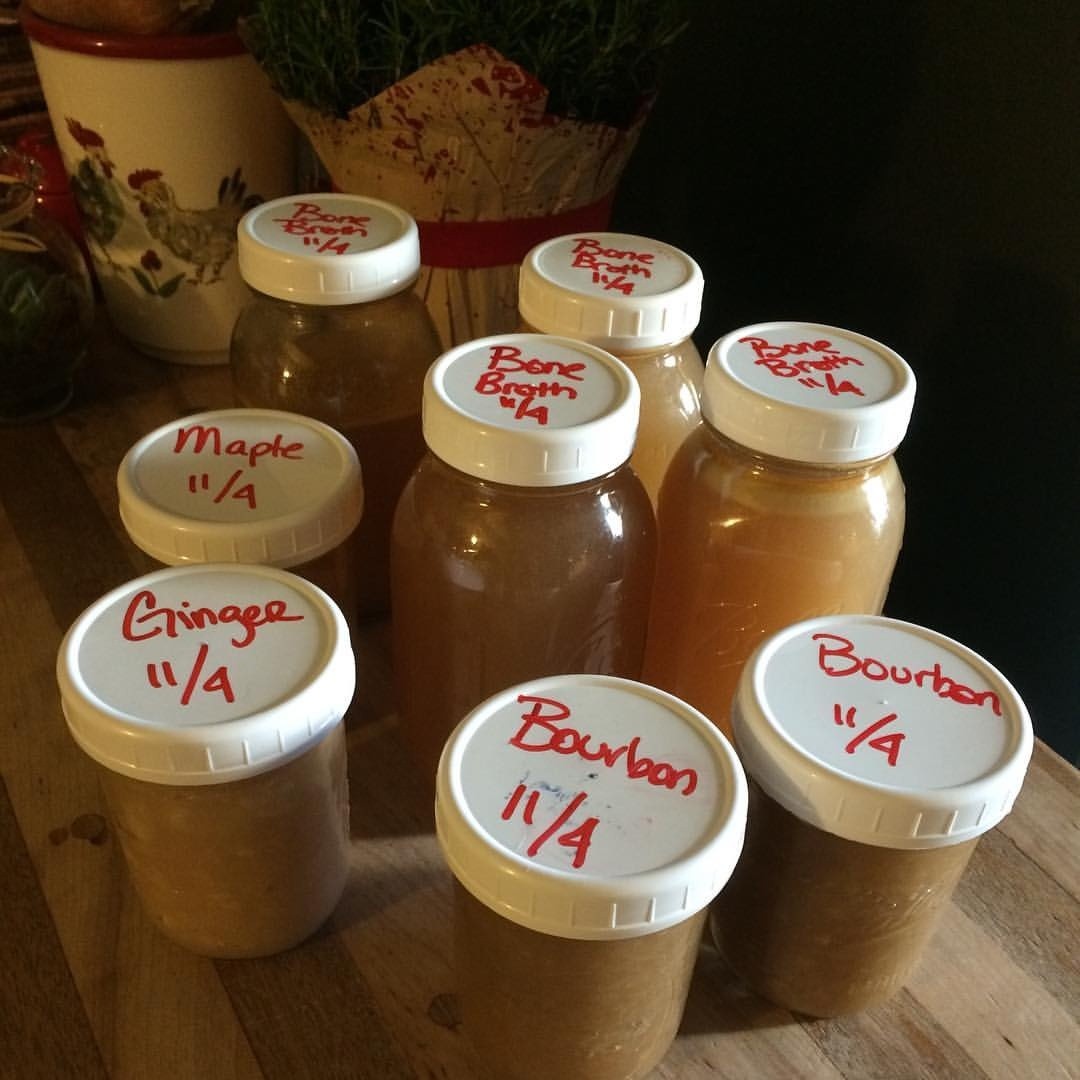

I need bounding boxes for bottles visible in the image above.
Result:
[56,193,1037,1079]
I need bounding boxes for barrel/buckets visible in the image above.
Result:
[16,6,322,365]
